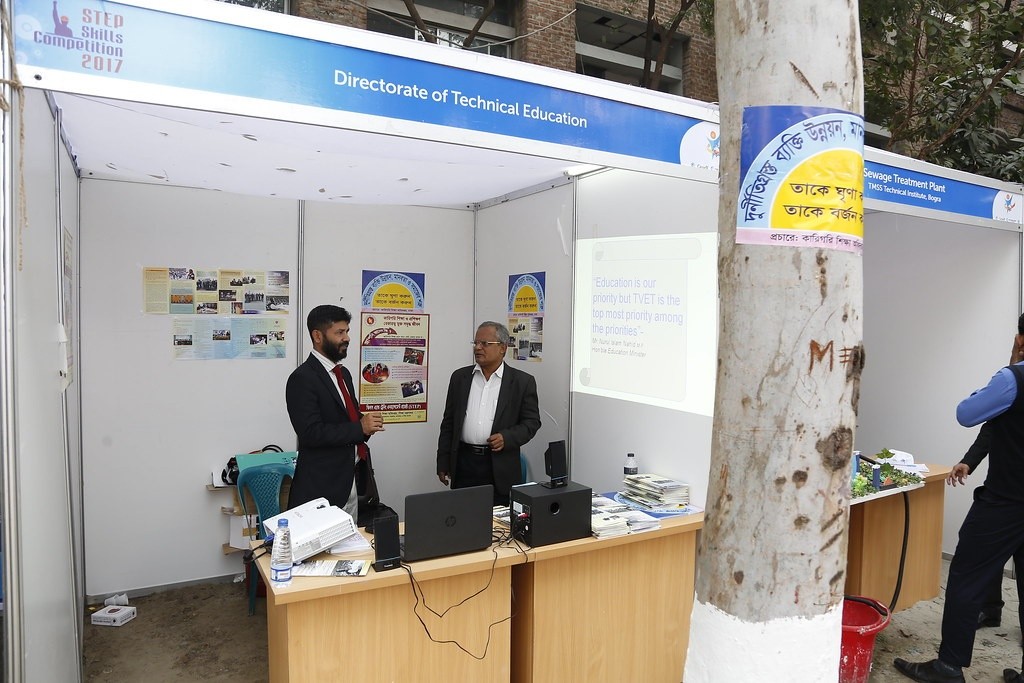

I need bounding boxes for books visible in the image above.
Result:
[590,473,689,540]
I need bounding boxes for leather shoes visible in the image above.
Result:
[1003,668,1021,683]
[894,658,966,683]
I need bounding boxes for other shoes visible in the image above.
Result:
[976,611,1001,629]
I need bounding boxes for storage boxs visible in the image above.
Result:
[229,514,259,549]
[262,497,356,564]
[91,605,137,626]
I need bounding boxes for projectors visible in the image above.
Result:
[263,497,356,565]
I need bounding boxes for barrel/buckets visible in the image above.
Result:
[838,596,890,683]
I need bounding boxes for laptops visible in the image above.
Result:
[400,485,495,562]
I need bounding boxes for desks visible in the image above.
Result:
[843,454,954,615]
[250,492,704,683]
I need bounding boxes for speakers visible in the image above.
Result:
[371,515,401,573]
[510,480,592,549]
[541,440,568,488]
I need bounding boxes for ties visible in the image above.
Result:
[333,365,367,459]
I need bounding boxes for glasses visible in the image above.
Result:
[471,340,500,347]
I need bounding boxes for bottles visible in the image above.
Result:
[624,453,638,478]
[270,519,292,588]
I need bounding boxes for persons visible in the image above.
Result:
[363,363,390,383]
[437,321,541,507]
[892,313,1024,683]
[287,304,385,511]
[401,381,423,397]
[187,269,289,314]
[404,348,424,364]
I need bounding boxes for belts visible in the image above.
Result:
[466,445,490,457]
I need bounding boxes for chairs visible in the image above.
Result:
[237,463,295,617]
